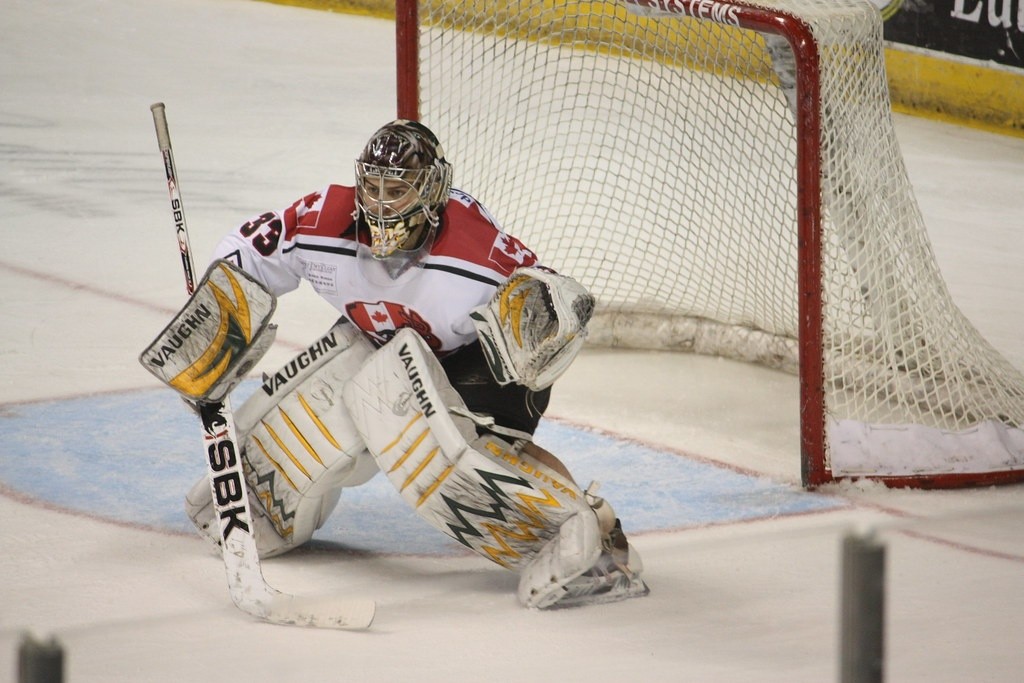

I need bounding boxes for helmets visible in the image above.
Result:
[354,120,450,182]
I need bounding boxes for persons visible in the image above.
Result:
[139,120,649,610]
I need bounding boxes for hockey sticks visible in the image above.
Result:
[149,100,379,632]
